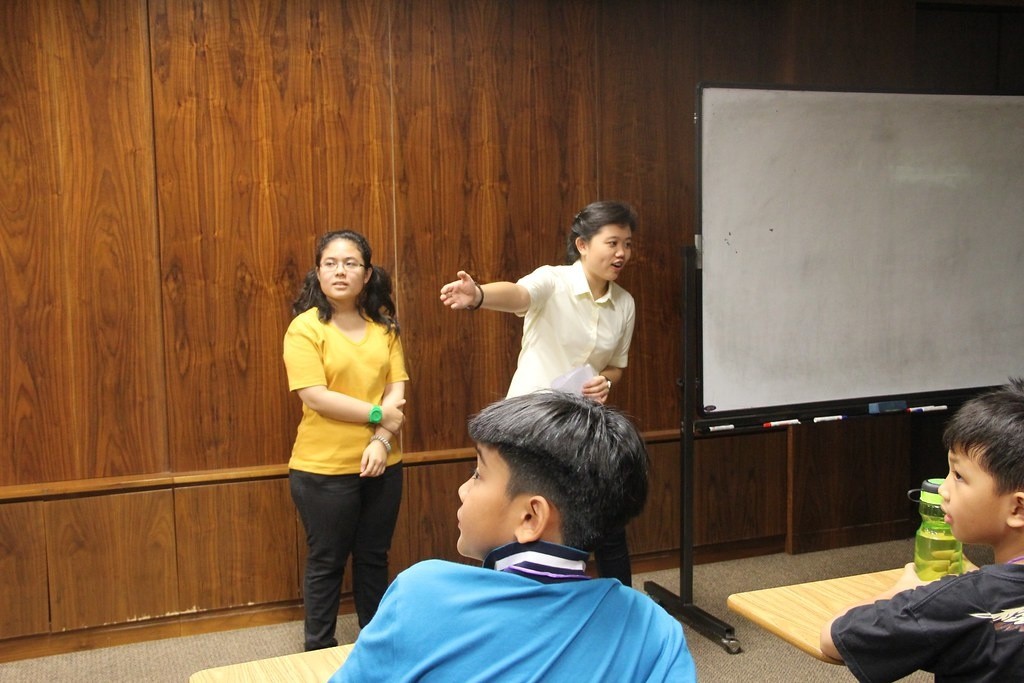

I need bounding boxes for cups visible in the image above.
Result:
[908,479,962,581]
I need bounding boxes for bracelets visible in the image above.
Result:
[467,285,484,310]
[606,377,612,388]
[370,435,391,451]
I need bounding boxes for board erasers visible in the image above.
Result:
[869,399,908,414]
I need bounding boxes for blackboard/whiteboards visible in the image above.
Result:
[702,86,1024,415]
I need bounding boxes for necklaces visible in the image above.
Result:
[1008,556,1024,563]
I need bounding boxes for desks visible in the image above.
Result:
[189,643,357,683]
[727,567,929,666]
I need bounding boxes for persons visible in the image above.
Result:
[821,378,1024,683]
[283,231,410,652]
[328,392,696,682]
[439,199,636,405]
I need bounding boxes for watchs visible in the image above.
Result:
[370,405,382,423]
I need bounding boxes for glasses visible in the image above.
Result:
[319,261,368,272]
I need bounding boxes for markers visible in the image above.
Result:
[698,423,738,435]
[906,403,948,414]
[761,413,851,429]
